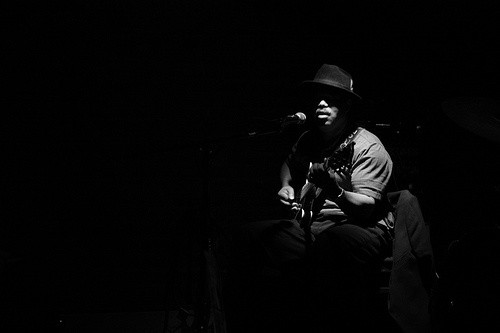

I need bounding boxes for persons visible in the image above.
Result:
[260,63,399,333]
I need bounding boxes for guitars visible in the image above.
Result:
[290,142,357,230]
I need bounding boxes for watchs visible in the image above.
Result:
[330,187,344,204]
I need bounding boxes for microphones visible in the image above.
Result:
[279,112,307,124]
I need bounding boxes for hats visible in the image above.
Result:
[302,63,363,101]
